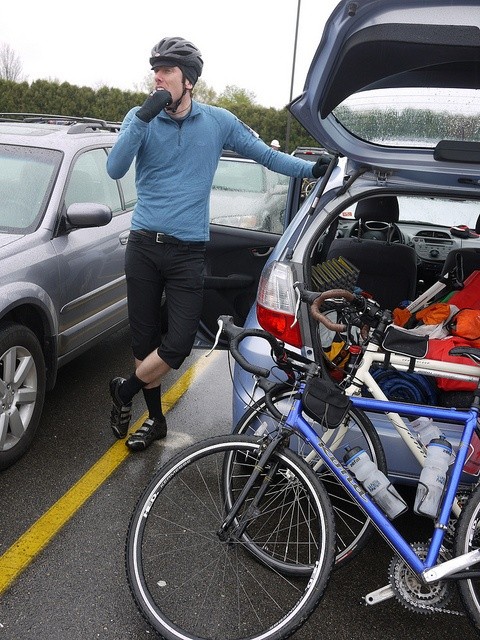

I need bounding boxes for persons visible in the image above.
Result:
[104,35,340,451]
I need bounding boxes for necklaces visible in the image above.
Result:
[162,102,193,116]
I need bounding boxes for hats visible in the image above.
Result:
[271,139,281,148]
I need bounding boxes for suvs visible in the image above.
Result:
[0,113,168,476]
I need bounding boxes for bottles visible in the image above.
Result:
[406,415,457,465]
[343,444,407,519]
[412,433,453,520]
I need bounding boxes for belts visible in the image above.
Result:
[137,231,185,244]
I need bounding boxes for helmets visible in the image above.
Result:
[149,37,205,77]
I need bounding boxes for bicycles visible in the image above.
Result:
[123,315,480,640]
[299,177,317,199]
[221,279,480,580]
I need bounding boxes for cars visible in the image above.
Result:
[197,1,480,495]
[208,157,291,234]
[290,146,331,163]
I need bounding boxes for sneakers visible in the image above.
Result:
[109,376,132,439]
[125,418,167,450]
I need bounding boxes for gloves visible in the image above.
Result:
[312,157,338,179]
[135,89,172,123]
